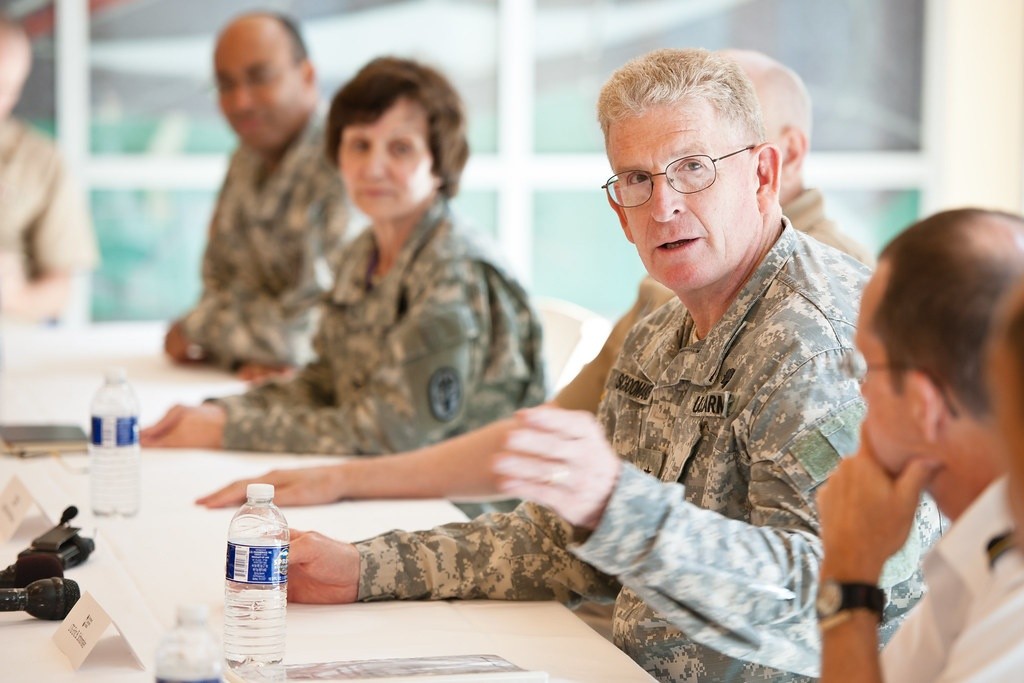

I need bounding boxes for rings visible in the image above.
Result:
[550,466,563,483]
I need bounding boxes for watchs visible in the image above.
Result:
[814,580,886,631]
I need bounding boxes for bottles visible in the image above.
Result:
[90,367,142,514]
[220,483,291,683]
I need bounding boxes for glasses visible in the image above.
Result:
[601,146,755,207]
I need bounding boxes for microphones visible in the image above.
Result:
[0,505,95,620]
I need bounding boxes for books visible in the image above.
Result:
[0,426,89,454]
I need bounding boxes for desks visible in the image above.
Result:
[0,321,661,683]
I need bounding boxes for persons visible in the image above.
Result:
[140,54,546,519]
[0,15,101,323]
[160,10,349,386]
[815,208,1023,683]
[195,49,877,509]
[230,49,942,683]
